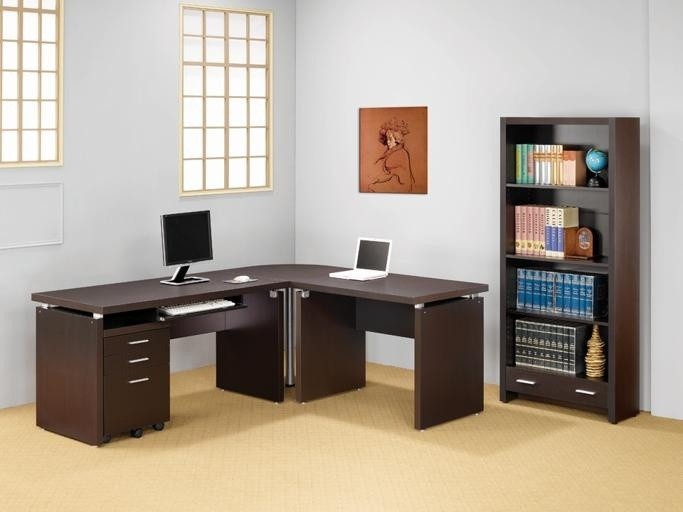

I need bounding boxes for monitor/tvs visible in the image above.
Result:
[159,209,214,286]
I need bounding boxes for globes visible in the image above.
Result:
[585,148,607,187]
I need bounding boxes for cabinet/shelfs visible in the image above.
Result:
[497,115,642,424]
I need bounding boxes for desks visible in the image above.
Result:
[32,263,488,447]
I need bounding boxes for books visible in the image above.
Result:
[513,143,587,186]
[514,205,579,261]
[516,267,602,377]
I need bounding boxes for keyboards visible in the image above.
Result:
[159,298,236,317]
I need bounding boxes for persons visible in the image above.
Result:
[366,116,416,192]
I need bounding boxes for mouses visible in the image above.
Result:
[234,275,250,282]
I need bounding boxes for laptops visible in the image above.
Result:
[329,237,392,282]
